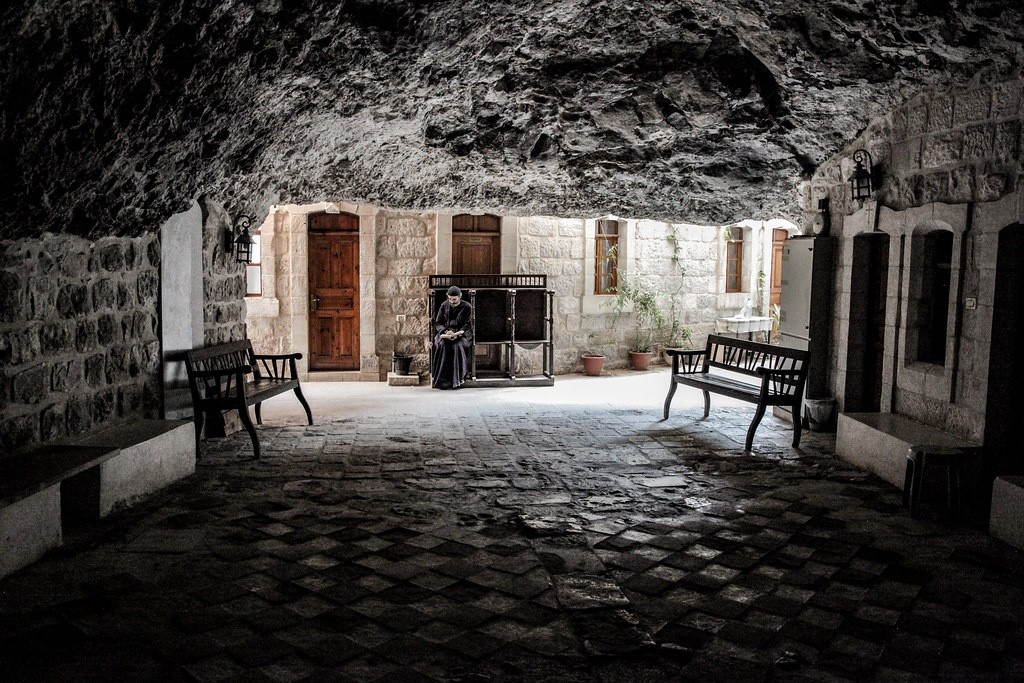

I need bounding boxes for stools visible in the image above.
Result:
[902,445,966,522]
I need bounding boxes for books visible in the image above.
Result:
[439,330,465,340]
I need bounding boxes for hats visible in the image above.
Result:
[445,286,461,296]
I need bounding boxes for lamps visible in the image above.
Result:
[225,214,256,265]
[848,149,877,206]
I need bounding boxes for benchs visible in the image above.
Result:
[663,333,812,451]
[184,338,314,460]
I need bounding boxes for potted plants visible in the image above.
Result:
[596,241,665,371]
[679,329,698,364]
[664,229,684,367]
[580,333,606,377]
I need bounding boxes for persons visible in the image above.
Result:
[430,285,473,391]
